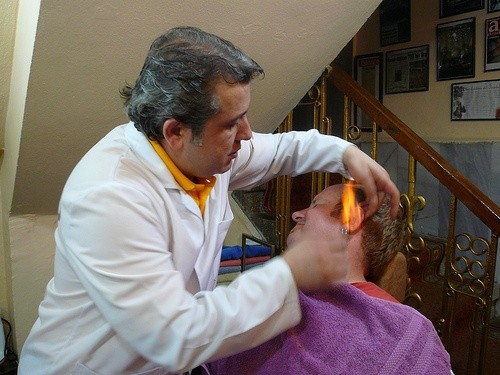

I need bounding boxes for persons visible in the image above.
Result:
[17,27,400,375]
[202,183,454,375]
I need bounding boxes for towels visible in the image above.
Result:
[195,283,450,375]
[216,244,271,287]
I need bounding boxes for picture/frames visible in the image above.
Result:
[354,0,500,133]
